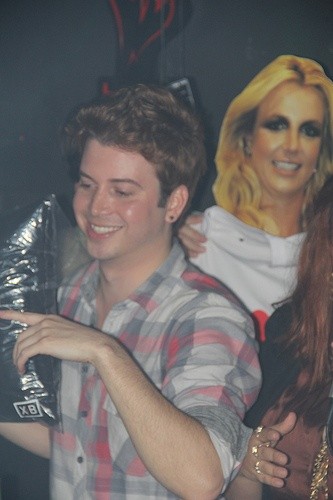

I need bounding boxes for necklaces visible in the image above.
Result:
[307,406,332,500]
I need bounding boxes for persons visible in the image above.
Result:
[0,81,264,500]
[186,52,333,350]
[56,211,207,282]
[221,181,333,500]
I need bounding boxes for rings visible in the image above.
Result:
[254,425,264,442]
[254,460,263,475]
[250,444,270,459]
